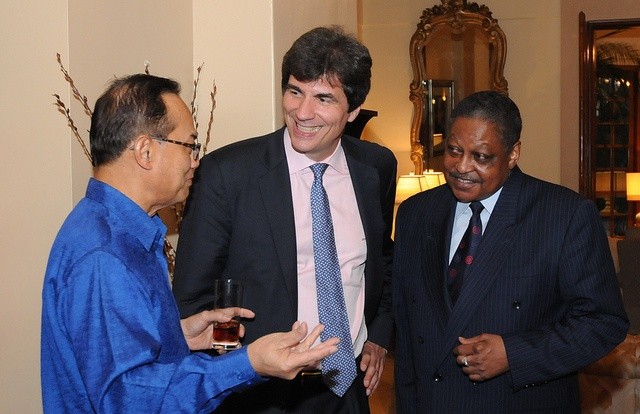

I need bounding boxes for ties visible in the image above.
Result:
[448,201,484,308]
[309,163,358,396]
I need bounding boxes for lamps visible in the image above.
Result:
[625,172,640,202]
[423,171,448,189]
[390,174,430,242]
[592,171,626,214]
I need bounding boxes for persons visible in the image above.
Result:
[171,27,397,413]
[393,91,640,413]
[39,73,339,414]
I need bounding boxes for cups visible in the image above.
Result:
[212,278,243,351]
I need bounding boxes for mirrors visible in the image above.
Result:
[577,10,640,236]
[429,78,455,158]
[407,0,510,176]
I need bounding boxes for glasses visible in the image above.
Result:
[129,136,201,160]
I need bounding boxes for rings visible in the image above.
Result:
[462,356,468,367]
